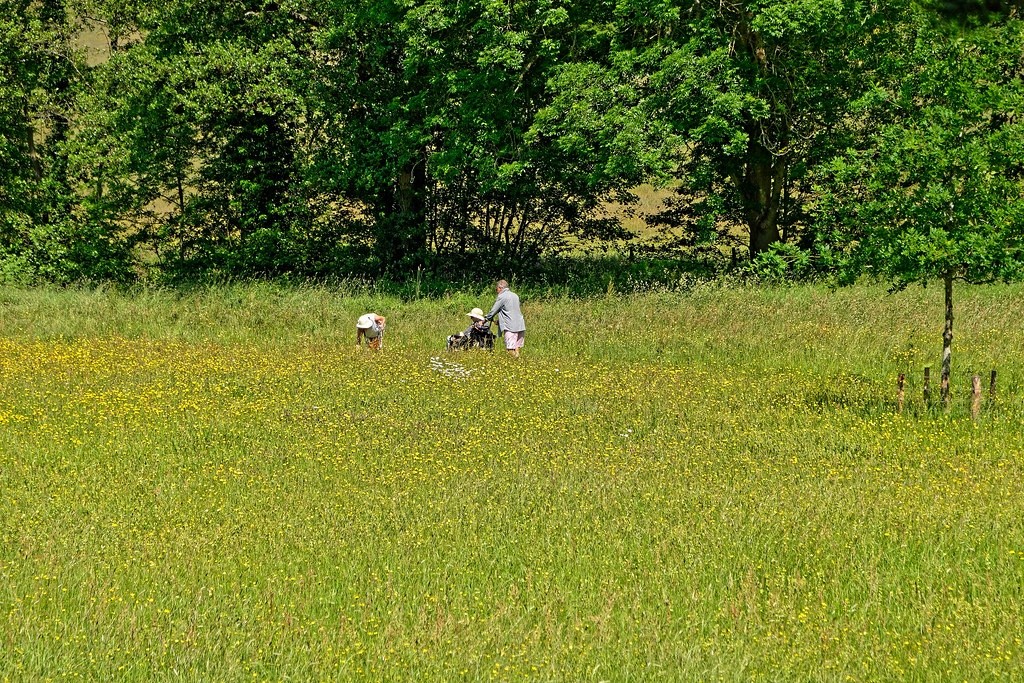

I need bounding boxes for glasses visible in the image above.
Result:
[470,317,474,319]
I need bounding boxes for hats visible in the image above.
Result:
[466,308,486,321]
[356,316,373,329]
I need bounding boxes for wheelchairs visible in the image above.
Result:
[460,319,497,353]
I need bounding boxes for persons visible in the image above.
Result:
[356,313,385,348]
[464,280,526,352]
